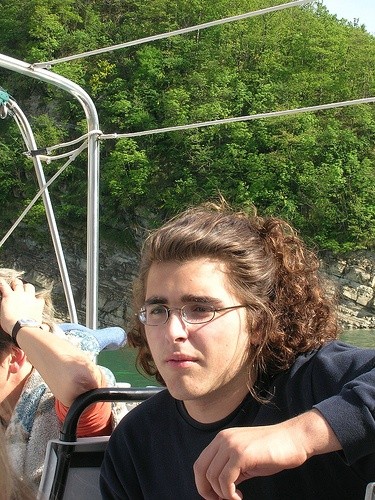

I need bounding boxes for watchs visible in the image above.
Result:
[12,318,43,349]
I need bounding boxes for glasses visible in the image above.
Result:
[134,303,245,326]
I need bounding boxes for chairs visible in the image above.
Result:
[36,387,169,500]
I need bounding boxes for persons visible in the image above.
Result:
[99,201,375,500]
[0,267,127,500]
